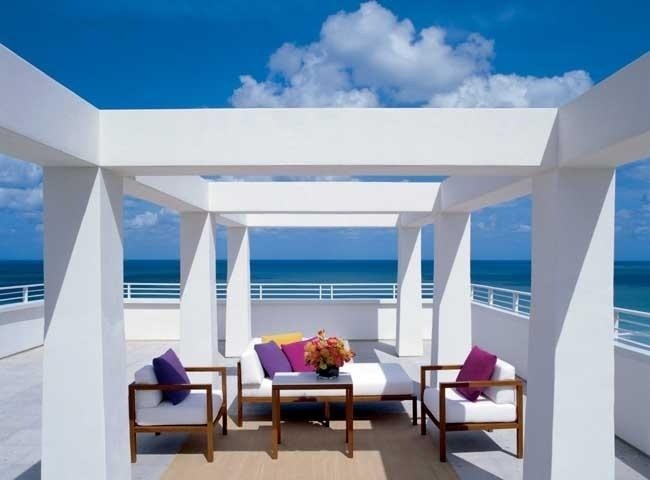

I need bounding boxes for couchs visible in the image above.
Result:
[237,338,418,428]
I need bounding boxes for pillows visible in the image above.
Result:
[454,345,497,402]
[254,332,353,385]
[152,348,191,406]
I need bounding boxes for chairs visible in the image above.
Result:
[126,365,229,464]
[419,357,525,464]
